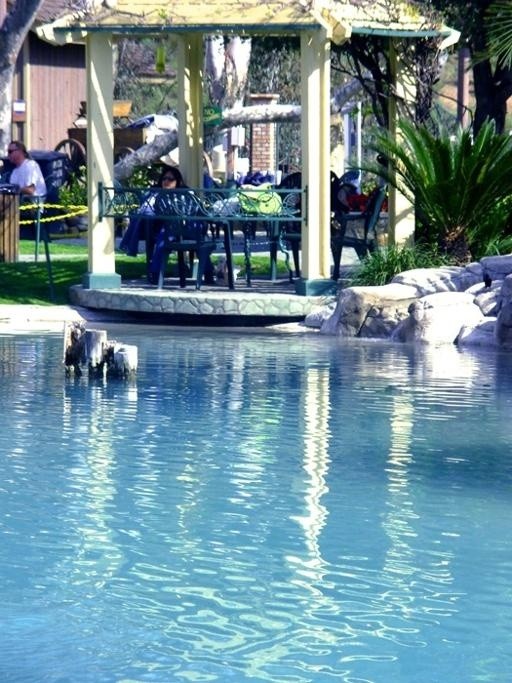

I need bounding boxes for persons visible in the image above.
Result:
[7,140,48,216]
[141,166,216,284]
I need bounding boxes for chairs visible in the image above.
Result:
[155,189,229,290]
[332,184,387,279]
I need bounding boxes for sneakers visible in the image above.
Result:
[147,272,159,285]
[205,275,217,286]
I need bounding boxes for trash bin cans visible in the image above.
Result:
[0,150,69,243]
[0,183,21,264]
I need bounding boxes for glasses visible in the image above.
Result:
[162,176,176,181]
[8,149,19,154]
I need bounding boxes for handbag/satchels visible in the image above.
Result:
[238,182,283,215]
